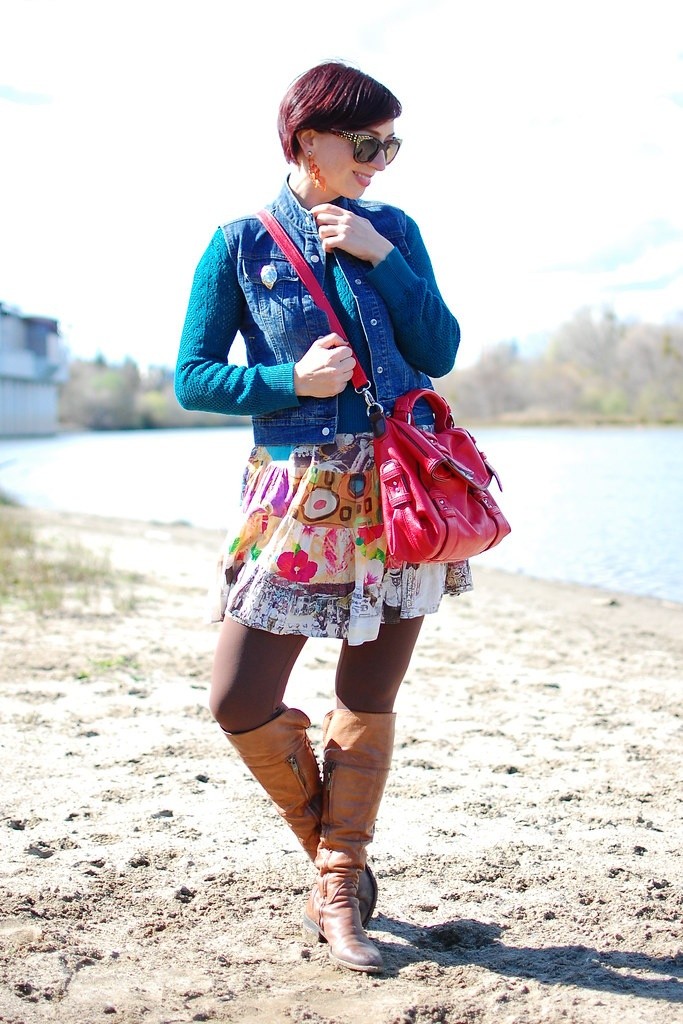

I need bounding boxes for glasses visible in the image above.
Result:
[324,126,402,165]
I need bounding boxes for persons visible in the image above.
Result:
[172,64,474,975]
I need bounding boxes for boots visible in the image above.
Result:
[218,708,378,928]
[302,709,397,973]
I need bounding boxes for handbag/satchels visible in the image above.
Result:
[369,389,511,563]
